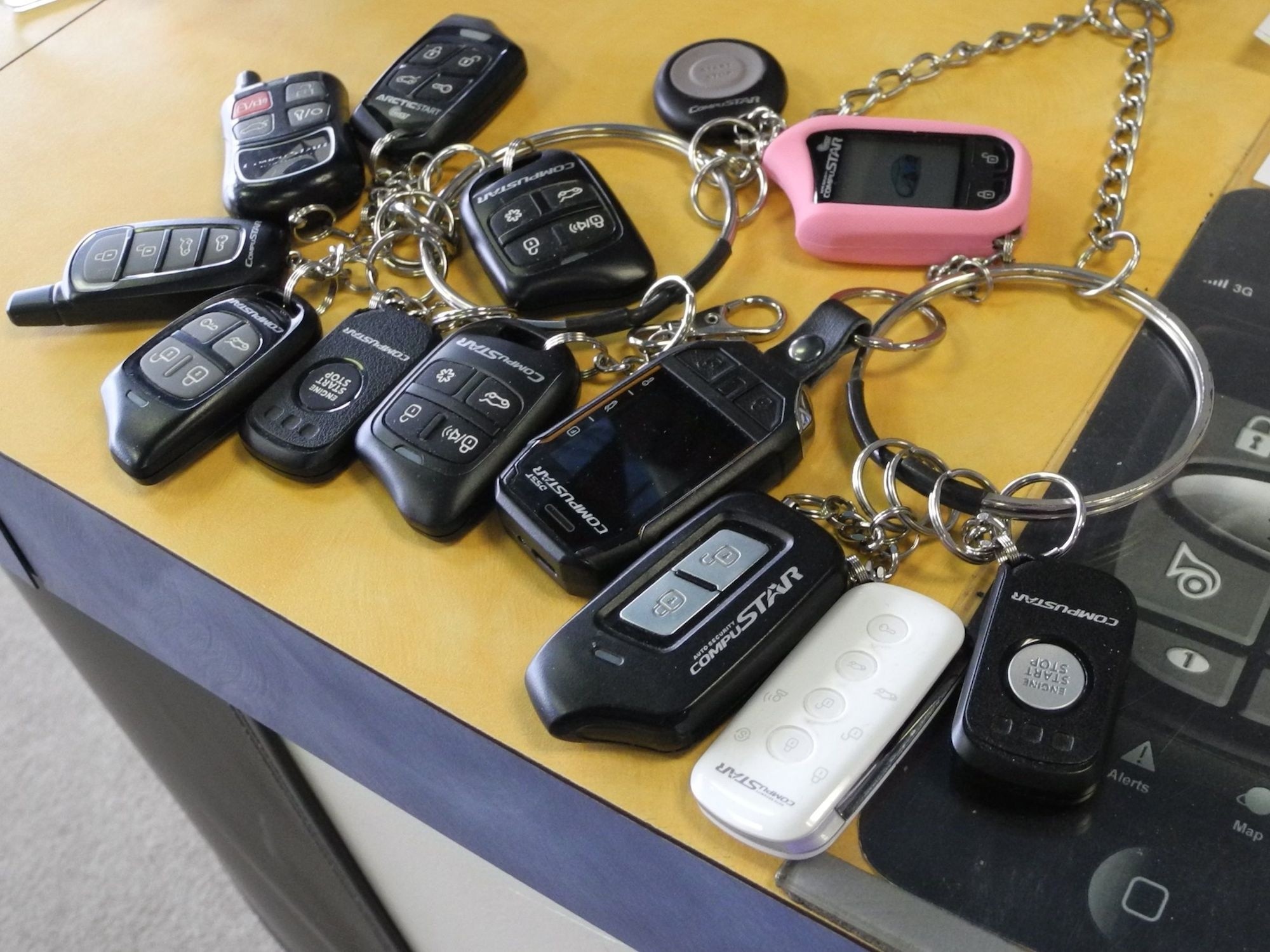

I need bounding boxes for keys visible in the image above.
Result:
[459,134,657,318]
[5,220,287,326]
[498,286,952,602]
[687,443,971,864]
[695,115,1030,272]
[222,62,365,241]
[930,469,1140,808]
[349,12,529,184]
[354,305,610,542]
[241,276,505,487]
[526,438,937,756]
[652,34,794,186]
[99,252,340,485]
